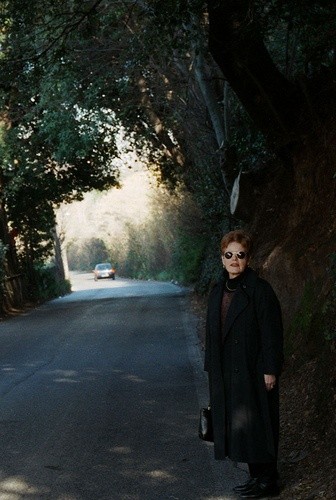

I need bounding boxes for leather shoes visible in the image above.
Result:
[239,481,280,498]
[233,478,258,492]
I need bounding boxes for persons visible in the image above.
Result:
[202,230,285,500]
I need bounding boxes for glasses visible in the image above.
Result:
[222,251,246,260]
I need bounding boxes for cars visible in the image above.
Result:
[93,262,115,281]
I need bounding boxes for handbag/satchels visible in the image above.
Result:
[200,407,214,441]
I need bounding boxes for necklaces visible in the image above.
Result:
[226,278,237,292]
